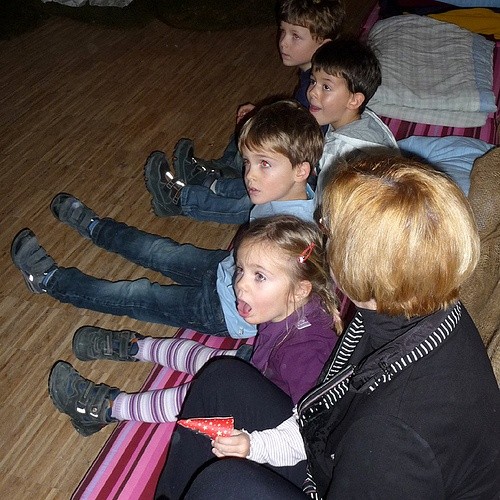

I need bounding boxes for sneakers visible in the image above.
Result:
[143,151,183,217]
[72,325,150,362]
[48,360,120,437]
[50,192,100,240]
[11,228,58,295]
[172,137,216,189]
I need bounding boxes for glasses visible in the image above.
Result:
[313,206,332,238]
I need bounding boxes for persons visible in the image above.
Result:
[203,0,347,181]
[148,152,500,500]
[144,35,403,224]
[10,99,320,340]
[48,214,344,467]
